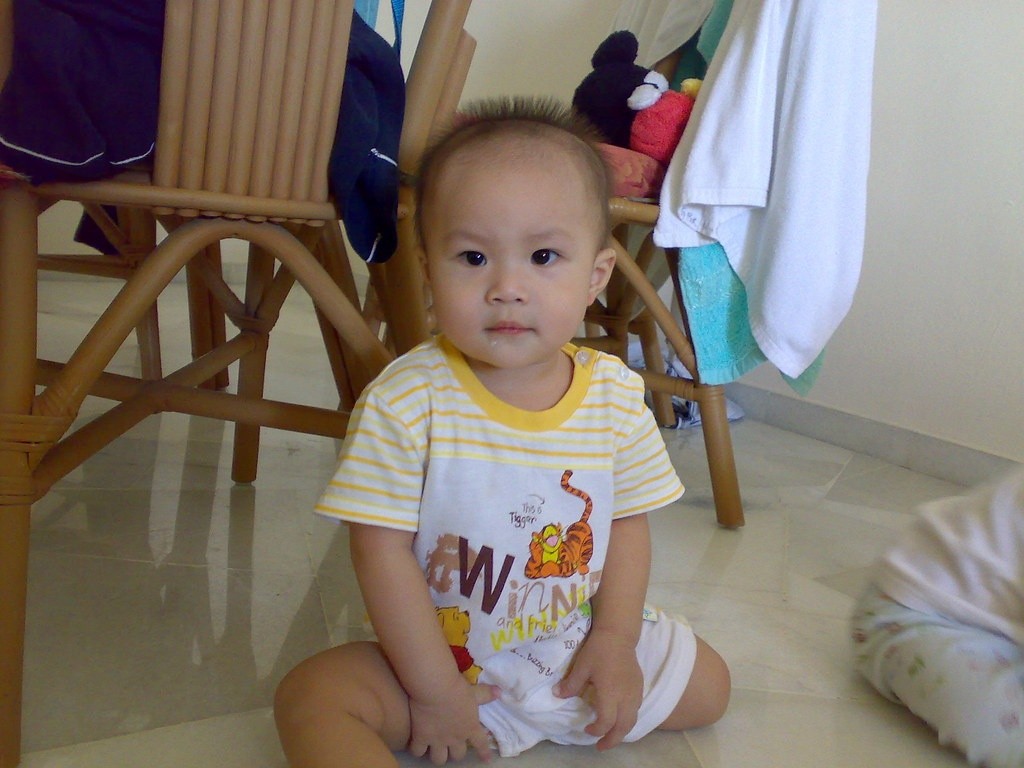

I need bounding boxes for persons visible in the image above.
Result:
[272,97,731,768]
[854,464,1024,767]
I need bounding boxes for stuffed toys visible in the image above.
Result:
[570,30,711,170]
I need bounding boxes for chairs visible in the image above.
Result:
[0,0,470,768]
[363,1,879,531]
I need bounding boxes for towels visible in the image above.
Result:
[653,0,879,397]
